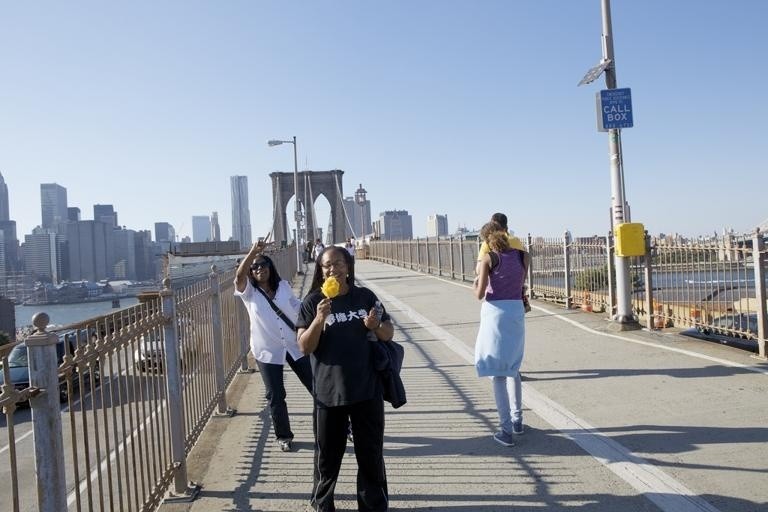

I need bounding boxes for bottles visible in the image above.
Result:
[366,301,384,341]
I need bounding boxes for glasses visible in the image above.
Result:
[250,261,269,270]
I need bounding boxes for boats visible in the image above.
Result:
[23,279,127,306]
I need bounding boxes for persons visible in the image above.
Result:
[295,245,394,512]
[232,231,354,451]
[471,220,531,449]
[344,236,356,265]
[301,237,325,263]
[475,214,525,276]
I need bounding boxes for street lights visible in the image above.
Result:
[355,183,368,244]
[268,135,303,276]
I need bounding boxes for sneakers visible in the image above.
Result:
[511,418,524,435]
[494,431,514,447]
[278,436,293,452]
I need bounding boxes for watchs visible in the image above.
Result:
[373,321,384,331]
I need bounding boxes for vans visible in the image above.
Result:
[134,318,198,373]
[0,326,100,409]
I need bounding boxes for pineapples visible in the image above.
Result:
[320,276,339,299]
